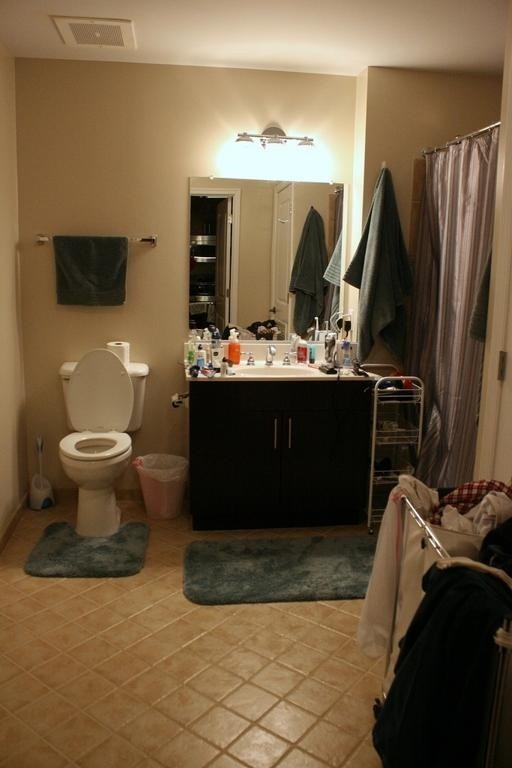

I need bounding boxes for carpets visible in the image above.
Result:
[22,520,149,578]
[182,536,376,605]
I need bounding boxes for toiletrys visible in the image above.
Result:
[228,328,236,339]
[309,345,316,363]
[186,327,229,378]
[228,331,241,364]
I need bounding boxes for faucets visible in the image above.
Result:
[266,345,276,365]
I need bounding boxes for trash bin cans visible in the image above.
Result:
[132,454,191,522]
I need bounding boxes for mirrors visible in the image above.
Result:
[188,176,345,341]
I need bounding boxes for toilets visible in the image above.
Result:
[57,348,150,537]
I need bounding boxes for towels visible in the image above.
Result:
[342,165,416,366]
[287,207,341,337]
[52,235,128,306]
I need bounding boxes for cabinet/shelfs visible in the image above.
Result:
[361,361,425,534]
[189,382,372,530]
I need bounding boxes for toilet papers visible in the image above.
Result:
[105,341,128,369]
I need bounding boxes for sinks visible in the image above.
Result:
[237,365,313,376]
